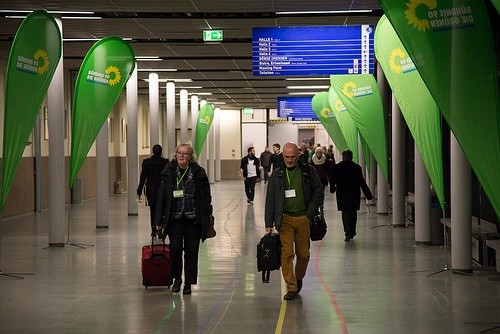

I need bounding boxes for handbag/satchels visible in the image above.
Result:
[206,216,216,237]
[257,232,280,272]
[310,217,327,240]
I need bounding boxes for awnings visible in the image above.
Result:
[310,91,348,155]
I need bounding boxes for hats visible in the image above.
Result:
[343,150,352,156]
[315,147,323,153]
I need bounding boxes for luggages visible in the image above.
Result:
[142,229,173,290]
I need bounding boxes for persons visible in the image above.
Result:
[155,144,213,295]
[265,142,325,301]
[330,149,374,242]
[261,144,283,184]
[298,135,335,186]
[137,144,170,239]
[239,147,263,205]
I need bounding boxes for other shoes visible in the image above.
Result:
[157,229,162,236]
[251,201,253,204]
[184,285,191,294]
[247,199,250,203]
[345,235,354,241]
[297,279,303,293]
[264,182,266,183]
[172,278,182,292]
[284,291,296,300]
[151,231,156,237]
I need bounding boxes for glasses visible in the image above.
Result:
[177,152,187,156]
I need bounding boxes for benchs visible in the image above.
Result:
[405,192,414,228]
[441,217,500,280]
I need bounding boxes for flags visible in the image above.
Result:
[1,9,61,217]
[380,0,500,230]
[328,72,388,180]
[194,102,215,163]
[372,13,445,210]
[70,35,136,190]
[325,86,358,164]
